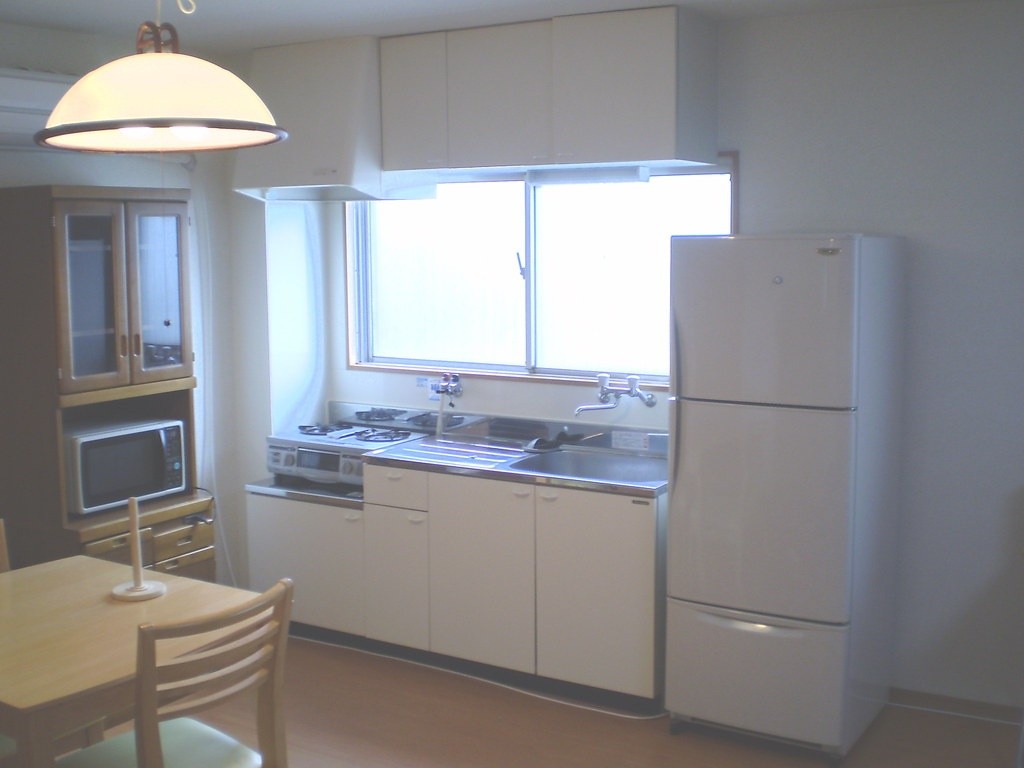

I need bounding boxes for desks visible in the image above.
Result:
[0,556,295,768]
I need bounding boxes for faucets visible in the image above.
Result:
[574,387,622,417]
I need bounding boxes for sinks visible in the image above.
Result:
[508,447,669,484]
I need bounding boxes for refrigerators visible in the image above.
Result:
[665,233,901,760]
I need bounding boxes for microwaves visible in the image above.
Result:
[64,420,187,515]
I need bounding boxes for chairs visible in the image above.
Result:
[55,576,294,768]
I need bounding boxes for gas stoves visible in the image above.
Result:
[265,400,465,484]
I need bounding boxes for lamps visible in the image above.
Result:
[32,0,289,154]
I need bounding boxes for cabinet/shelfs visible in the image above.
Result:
[379,30,450,173]
[445,4,718,174]
[426,470,667,717]
[245,465,431,666]
[0,185,219,586]
